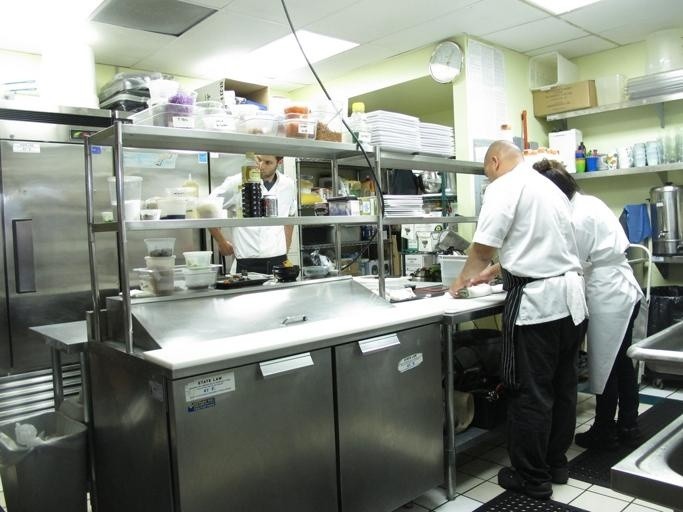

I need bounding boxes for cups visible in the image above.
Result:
[574,140,662,174]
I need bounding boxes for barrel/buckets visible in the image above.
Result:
[438,253,469,288]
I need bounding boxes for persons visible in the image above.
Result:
[445,138,591,502]
[540,167,648,450]
[196,153,299,276]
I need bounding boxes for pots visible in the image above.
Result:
[646,181,683,257]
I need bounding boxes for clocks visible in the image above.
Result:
[428,39,463,85]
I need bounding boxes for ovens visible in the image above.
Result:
[0,116,130,381]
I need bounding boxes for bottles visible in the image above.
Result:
[347,101,369,144]
[239,151,264,218]
[182,171,199,220]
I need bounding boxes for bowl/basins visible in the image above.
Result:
[271,264,329,283]
[439,254,471,287]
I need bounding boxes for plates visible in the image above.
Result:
[342,109,456,159]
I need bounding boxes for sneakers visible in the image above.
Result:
[575,419,618,448]
[498,467,553,498]
[552,470,568,484]
[618,418,640,447]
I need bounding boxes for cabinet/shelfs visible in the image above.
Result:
[82,118,483,352]
[546,90,682,179]
[296,156,456,281]
[0,137,299,379]
[86,324,446,511]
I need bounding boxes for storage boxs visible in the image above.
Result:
[193,77,270,113]
[532,79,596,118]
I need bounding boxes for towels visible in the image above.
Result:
[618,203,652,245]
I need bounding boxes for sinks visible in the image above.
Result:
[625,319,683,364]
[611,415,682,486]
